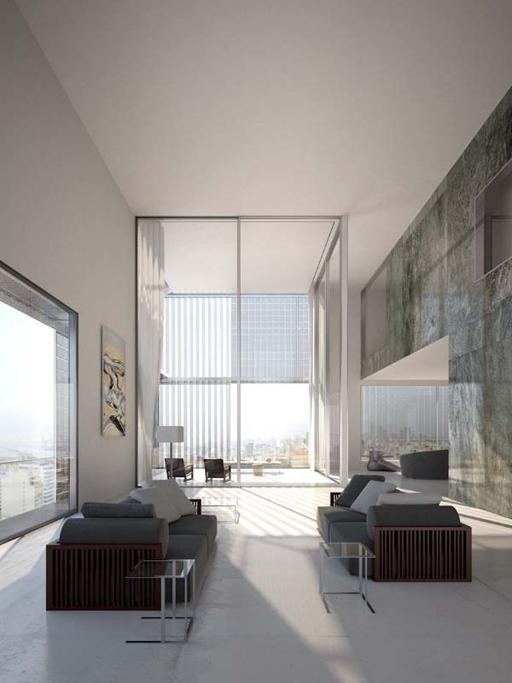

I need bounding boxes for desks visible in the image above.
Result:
[316,540,375,615]
[124,558,193,644]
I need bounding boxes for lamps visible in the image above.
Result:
[155,424,184,478]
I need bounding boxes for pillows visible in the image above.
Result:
[350,478,442,517]
[130,479,195,523]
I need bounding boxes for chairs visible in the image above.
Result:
[164,458,192,480]
[203,458,231,483]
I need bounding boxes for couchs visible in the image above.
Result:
[316,473,472,583]
[46,497,216,611]
